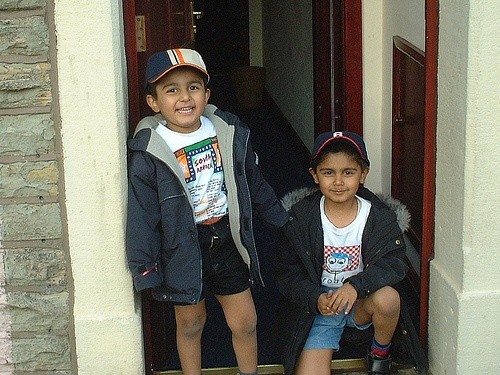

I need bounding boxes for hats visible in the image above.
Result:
[146,49,210,84]
[312,131,370,166]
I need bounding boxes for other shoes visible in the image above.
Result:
[366,342,394,375]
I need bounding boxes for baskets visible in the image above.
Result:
[230,66,266,102]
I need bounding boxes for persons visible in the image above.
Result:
[124,48,290,375]
[277,132,409,375]
